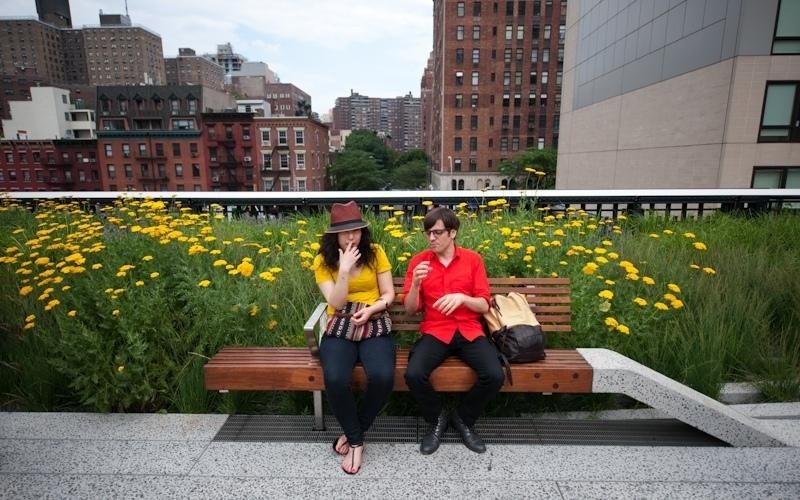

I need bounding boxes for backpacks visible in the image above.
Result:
[483,292,545,363]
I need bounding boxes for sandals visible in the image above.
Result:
[334,433,349,455]
[342,439,364,473]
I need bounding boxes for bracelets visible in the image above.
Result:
[374,298,389,308]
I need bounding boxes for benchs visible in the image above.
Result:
[205,277,592,431]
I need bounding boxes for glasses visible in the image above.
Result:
[421,229,446,238]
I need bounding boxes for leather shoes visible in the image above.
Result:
[449,410,485,452]
[420,408,448,455]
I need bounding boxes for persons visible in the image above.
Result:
[400,206,506,456]
[312,200,396,476]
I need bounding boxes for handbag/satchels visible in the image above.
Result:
[327,301,392,341]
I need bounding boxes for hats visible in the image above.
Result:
[324,201,371,234]
